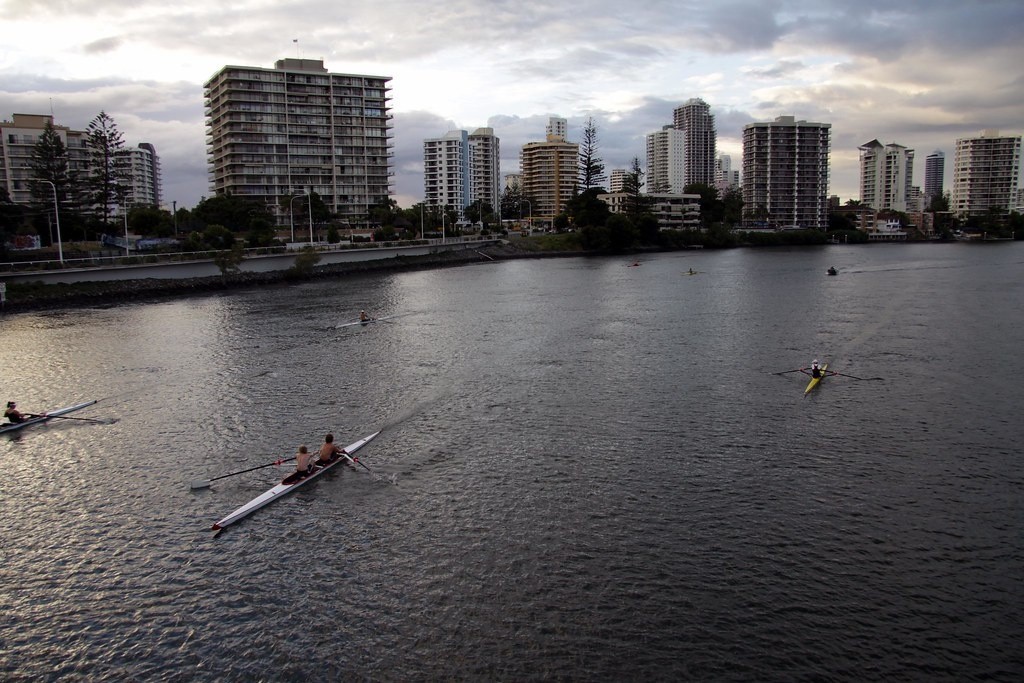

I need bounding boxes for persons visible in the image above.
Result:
[360,310,370,321]
[319,434,345,464]
[3,401,30,424]
[811,360,822,379]
[293,445,315,478]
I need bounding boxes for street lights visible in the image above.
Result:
[522,200,531,235]
[290,194,307,243]
[124,193,138,256]
[41,181,63,264]
[421,201,425,239]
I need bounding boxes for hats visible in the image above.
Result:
[813,359,819,362]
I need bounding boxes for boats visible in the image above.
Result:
[212,428,382,530]
[0,400,97,433]
[804,364,827,395]
[327,316,395,330]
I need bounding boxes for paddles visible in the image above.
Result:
[349,317,360,321]
[370,317,392,323]
[342,449,368,470]
[820,369,869,380]
[24,413,112,424]
[190,457,297,489]
[769,367,812,375]
